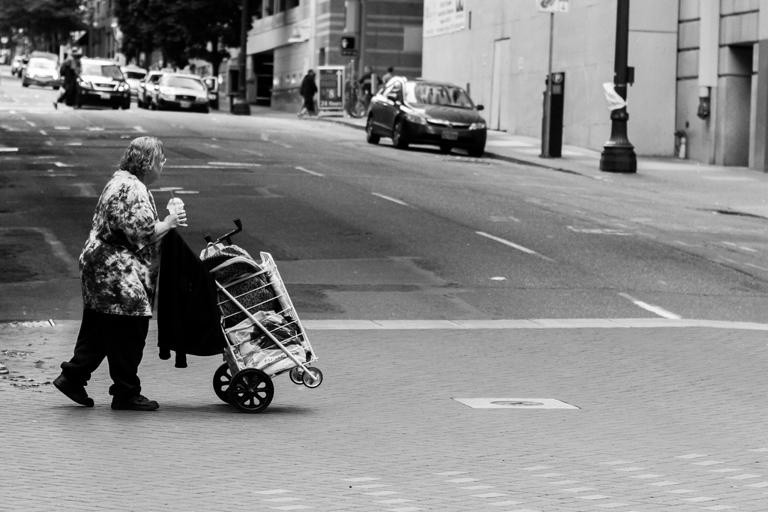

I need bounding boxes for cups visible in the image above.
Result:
[166,198,185,215]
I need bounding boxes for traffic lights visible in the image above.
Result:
[340,36,354,50]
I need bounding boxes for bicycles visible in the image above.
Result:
[346,79,378,119]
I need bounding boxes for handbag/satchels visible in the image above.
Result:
[211,245,275,325]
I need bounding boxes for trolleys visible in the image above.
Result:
[159,214,328,413]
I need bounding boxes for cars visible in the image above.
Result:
[361,75,488,158]
[6,51,162,111]
[151,74,210,113]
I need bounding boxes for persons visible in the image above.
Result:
[382,66,394,83]
[54,136,188,412]
[53,52,84,109]
[301,69,318,115]
[358,64,382,106]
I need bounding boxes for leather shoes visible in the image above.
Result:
[53,374,94,407]
[111,395,159,411]
